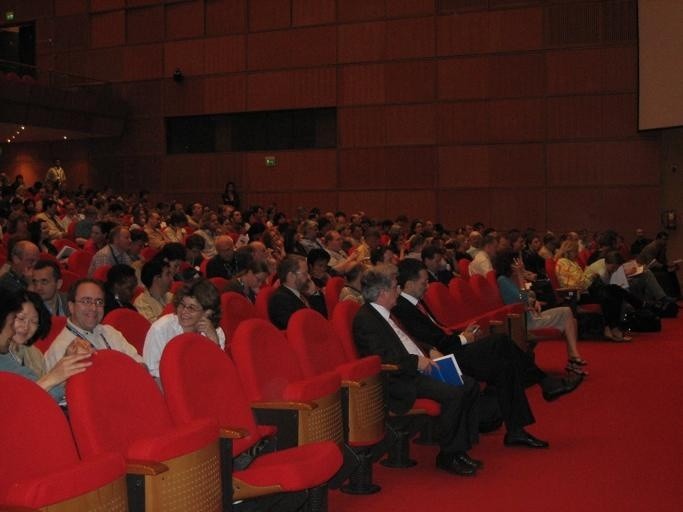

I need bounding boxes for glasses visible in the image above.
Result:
[74,299,105,307]
[175,303,201,313]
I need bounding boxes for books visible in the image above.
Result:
[423,353,465,385]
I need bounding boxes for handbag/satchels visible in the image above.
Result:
[624,311,661,333]
[576,310,605,341]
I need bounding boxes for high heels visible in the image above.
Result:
[569,356,589,368]
[566,366,589,376]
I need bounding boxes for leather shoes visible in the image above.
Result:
[613,333,632,341]
[456,452,482,468]
[542,374,583,401]
[435,450,476,476]
[603,336,623,342]
[504,429,549,448]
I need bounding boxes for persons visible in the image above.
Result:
[43,279,142,382]
[490,249,589,376]
[142,279,226,394]
[390,257,584,447]
[2,160,682,341]
[8,292,47,377]
[0,282,93,408]
[351,264,483,475]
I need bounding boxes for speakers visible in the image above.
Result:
[172,69,183,82]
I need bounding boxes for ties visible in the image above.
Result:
[415,301,427,316]
